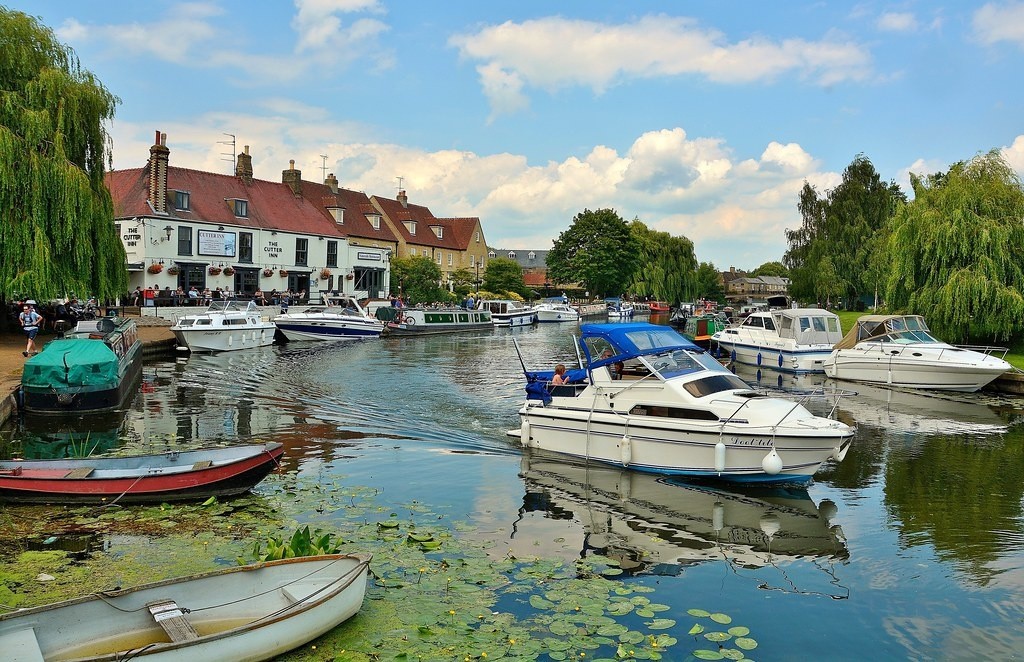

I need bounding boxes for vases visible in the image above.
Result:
[170,272,179,275]
[348,276,352,280]
[321,275,329,279]
[265,273,287,277]
[152,271,160,274]
[211,271,234,276]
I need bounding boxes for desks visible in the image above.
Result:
[275,294,301,305]
[196,294,206,306]
[234,294,245,301]
[172,293,186,307]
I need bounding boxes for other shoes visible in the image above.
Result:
[31,351,38,356]
[22,351,29,357]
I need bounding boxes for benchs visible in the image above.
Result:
[153,293,304,306]
[190,459,212,471]
[66,467,95,479]
[148,599,198,642]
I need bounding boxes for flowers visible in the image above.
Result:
[320,268,331,275]
[347,274,355,277]
[168,267,180,273]
[148,266,162,272]
[264,269,288,275]
[209,267,236,275]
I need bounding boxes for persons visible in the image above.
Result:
[704,300,711,313]
[189,287,204,306]
[255,288,268,306]
[19,301,56,356]
[131,284,186,307]
[477,296,483,310]
[216,286,236,307]
[71,300,95,318]
[552,364,569,386]
[271,289,306,306]
[203,288,213,307]
[391,296,400,308]
[562,292,569,311]
[467,295,474,310]
[602,351,624,380]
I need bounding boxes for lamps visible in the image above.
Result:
[273,264,277,270]
[273,232,278,236]
[219,260,224,268]
[161,226,174,241]
[312,266,317,272]
[319,237,324,241]
[218,226,225,230]
[159,257,165,265]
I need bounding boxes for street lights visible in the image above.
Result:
[475,261,482,300]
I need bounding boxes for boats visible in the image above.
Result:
[273,295,387,343]
[0,550,375,661]
[0,440,288,508]
[518,451,852,585]
[606,305,634,318]
[532,302,580,324]
[506,322,859,486]
[16,314,144,436]
[376,294,495,338]
[824,311,1014,395]
[710,306,847,374]
[167,299,279,354]
[667,307,687,330]
[476,298,537,328]
[822,378,1009,461]
